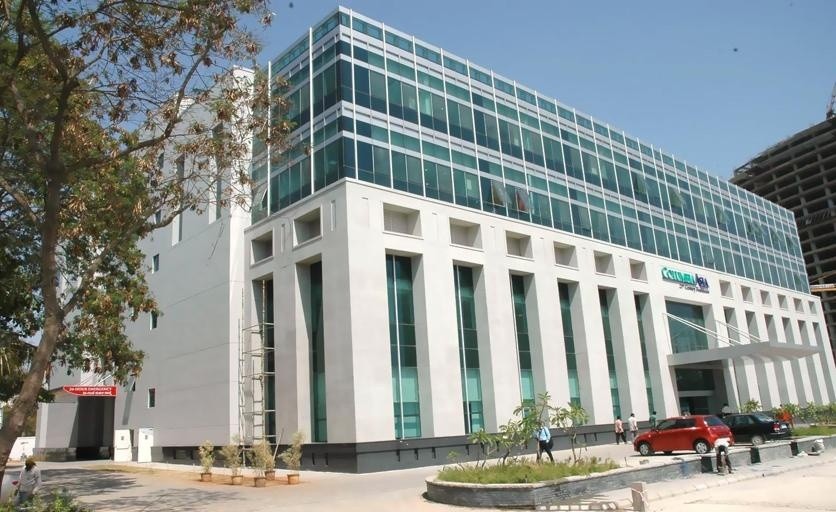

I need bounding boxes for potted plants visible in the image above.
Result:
[198,430,306,487]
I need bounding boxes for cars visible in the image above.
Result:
[633,414,735,456]
[716,412,792,445]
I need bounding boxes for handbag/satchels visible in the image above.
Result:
[547,438,553,449]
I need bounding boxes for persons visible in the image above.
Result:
[628,412,638,442]
[533,421,554,464]
[14,457,40,505]
[613,416,626,445]
[649,411,657,431]
[722,403,733,417]
[715,438,732,474]
